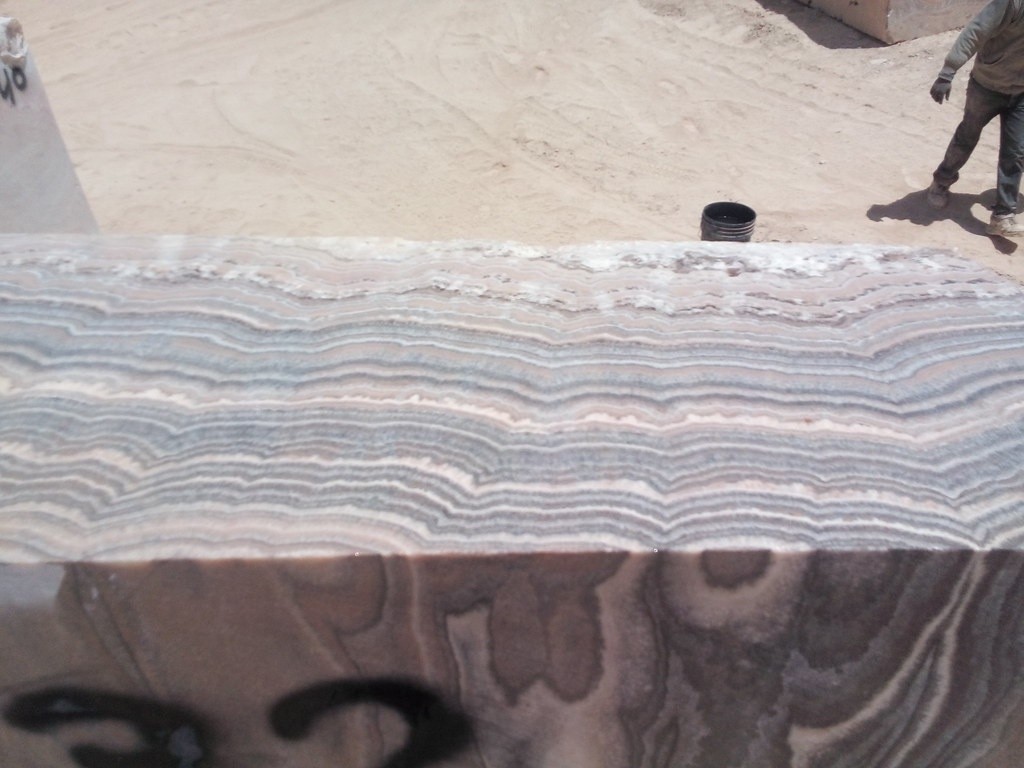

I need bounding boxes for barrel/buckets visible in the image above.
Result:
[699,202,757,242]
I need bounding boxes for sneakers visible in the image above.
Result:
[929,181,949,209]
[986,213,1023,237]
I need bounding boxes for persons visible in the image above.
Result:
[927,0,1024,239]
